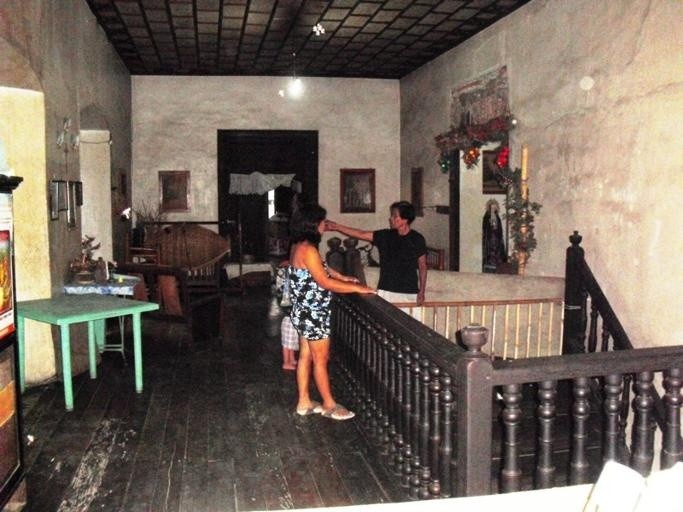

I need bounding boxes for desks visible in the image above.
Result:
[62,276,139,359]
[16,292,159,411]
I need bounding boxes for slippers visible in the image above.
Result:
[296,402,322,415]
[321,403,355,421]
[282,364,294,369]
[293,360,299,364]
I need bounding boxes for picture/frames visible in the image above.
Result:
[158,170,192,213]
[339,168,376,213]
[481,150,508,194]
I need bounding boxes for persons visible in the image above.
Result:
[287,204,377,421]
[280,261,299,370]
[324,201,427,323]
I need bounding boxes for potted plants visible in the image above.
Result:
[70,236,101,284]
[496,242,514,273]
